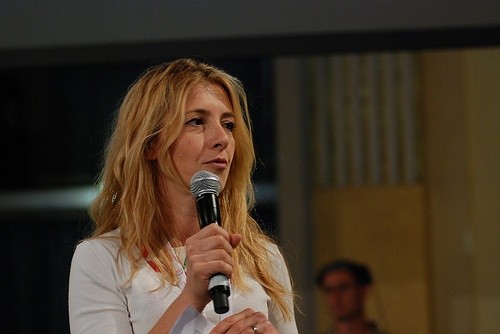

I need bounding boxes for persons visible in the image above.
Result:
[69,59,299,334]
[314,261,386,334]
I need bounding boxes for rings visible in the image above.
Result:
[249,325,259,334]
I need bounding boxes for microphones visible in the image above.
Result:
[190,170,231,314]
